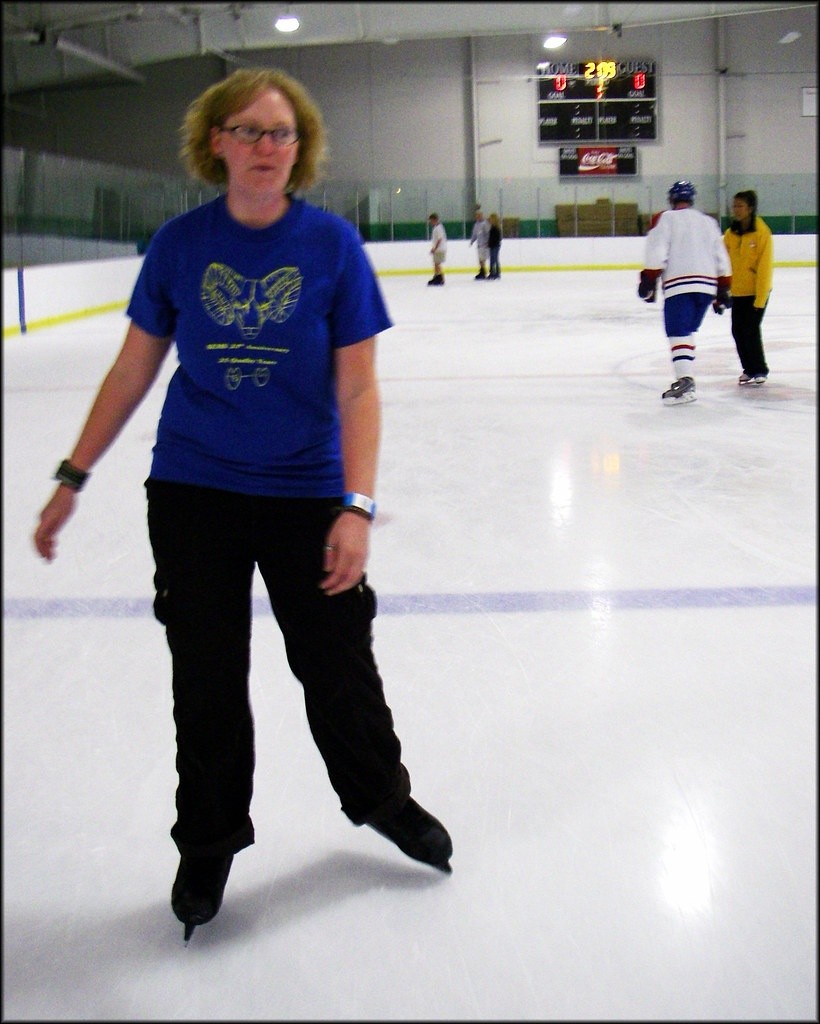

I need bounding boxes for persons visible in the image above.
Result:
[638,181,732,400]
[470,211,492,279]
[34,68,453,946]
[723,190,773,386]
[486,213,502,279]
[428,213,447,285]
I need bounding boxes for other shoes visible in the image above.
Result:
[475,269,486,280]
[428,274,443,285]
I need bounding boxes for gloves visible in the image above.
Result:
[638,271,656,302]
[716,285,729,315]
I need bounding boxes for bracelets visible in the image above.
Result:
[56,460,91,491]
[329,492,377,520]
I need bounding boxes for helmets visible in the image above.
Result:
[670,181,696,201]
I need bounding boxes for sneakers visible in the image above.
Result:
[170,851,234,942]
[738,374,753,385]
[755,377,766,384]
[365,796,453,874]
[663,377,697,406]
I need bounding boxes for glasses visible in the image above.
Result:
[220,124,302,146]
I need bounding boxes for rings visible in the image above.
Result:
[322,545,337,551]
